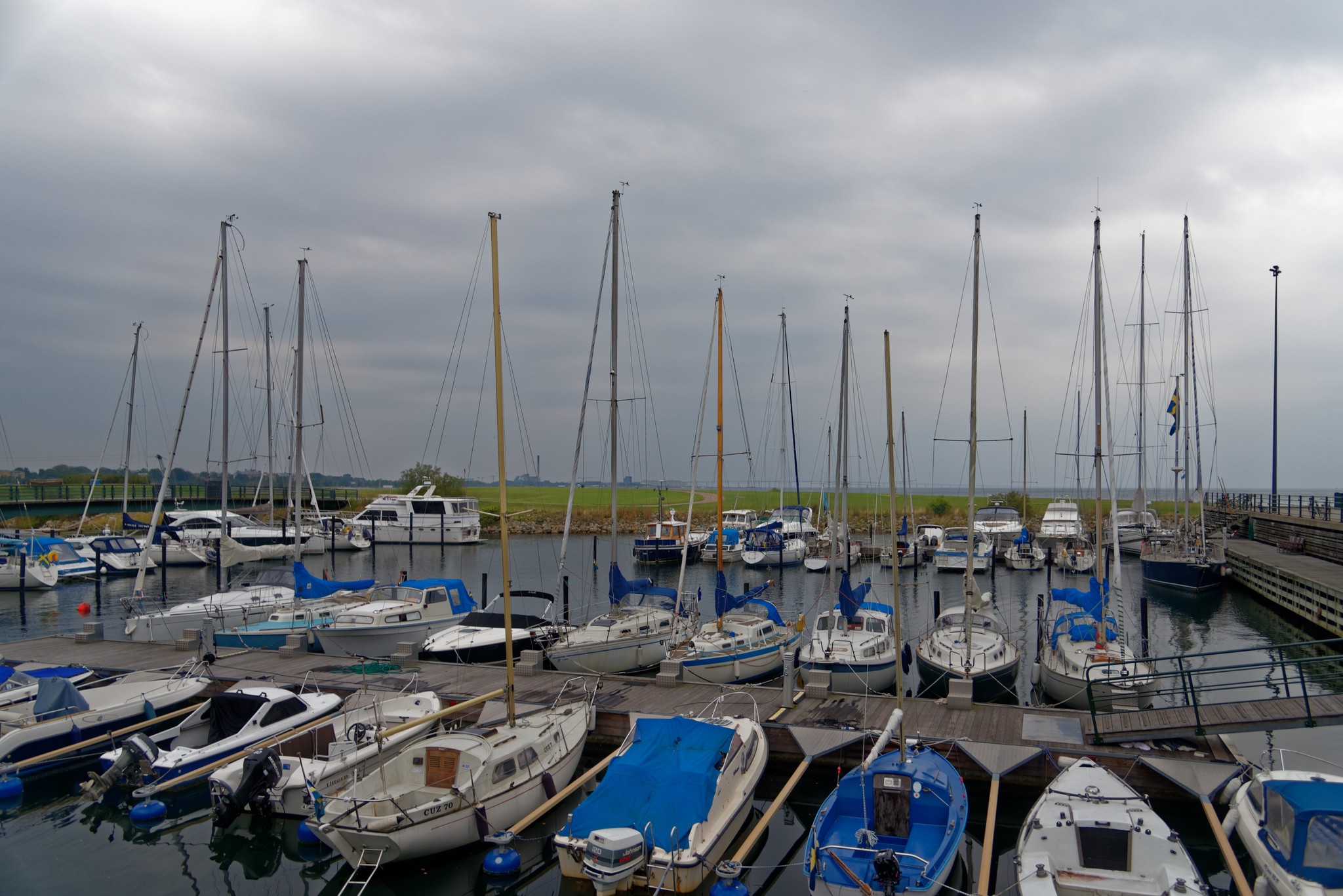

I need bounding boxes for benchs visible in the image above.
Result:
[1277,535,1306,555]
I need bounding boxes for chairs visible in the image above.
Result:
[328,740,357,761]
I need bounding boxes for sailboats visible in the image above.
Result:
[913,197,1023,706]
[654,270,803,687]
[795,295,912,696]
[201,246,404,656]
[536,184,700,680]
[117,211,309,647]
[302,207,599,871]
[0,188,1343,596]
[1028,204,1159,711]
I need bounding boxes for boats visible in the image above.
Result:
[550,689,769,896]
[0,661,94,707]
[420,589,579,665]
[802,730,969,896]
[631,478,709,566]
[1229,748,1343,896]
[304,575,477,661]
[203,688,443,839]
[0,655,209,812]
[74,680,345,833]
[1011,756,1211,896]
[303,475,489,545]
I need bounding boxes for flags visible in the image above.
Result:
[1167,387,1179,436]
